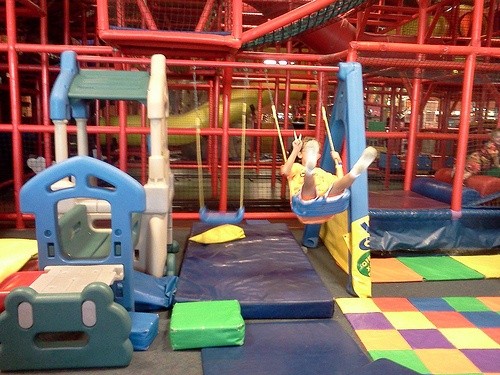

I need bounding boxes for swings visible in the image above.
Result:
[262,69,351,215]
[193,69,250,224]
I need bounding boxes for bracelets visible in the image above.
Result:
[336,161,342,166]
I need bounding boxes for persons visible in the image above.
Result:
[110,137,119,151]
[450,131,500,186]
[280,130,377,215]
